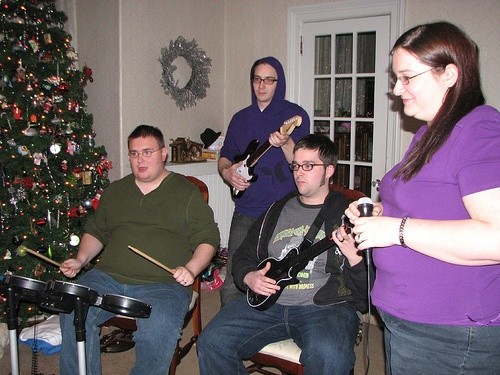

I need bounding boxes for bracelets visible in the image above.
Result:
[398,215,409,248]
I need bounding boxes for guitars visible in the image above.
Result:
[246,222,354,312]
[230,113,304,198]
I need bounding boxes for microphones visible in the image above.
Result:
[357,197,374,266]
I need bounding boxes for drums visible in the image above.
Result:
[1,274,46,303]
[100,293,152,320]
[50,279,98,304]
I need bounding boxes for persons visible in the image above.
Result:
[197,135,377,375]
[218,54,310,310]
[60,124,221,375]
[344,22,500,375]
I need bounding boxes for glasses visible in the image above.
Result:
[389,64,447,88]
[128,146,166,158]
[289,163,329,171]
[251,77,278,85]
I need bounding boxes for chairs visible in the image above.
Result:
[99,175,209,375]
[243,185,371,375]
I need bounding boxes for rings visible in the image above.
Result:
[357,233,360,240]
[181,278,186,284]
